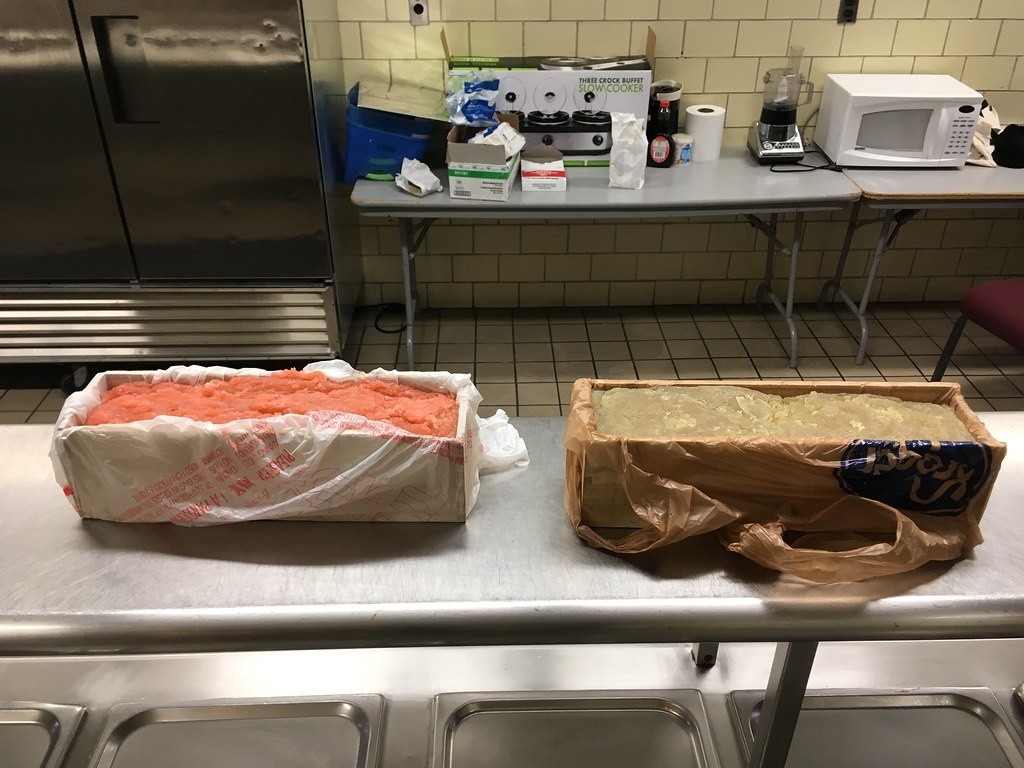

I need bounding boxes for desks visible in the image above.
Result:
[818,155,1024,364]
[351,145,862,370]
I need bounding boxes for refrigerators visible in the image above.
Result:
[0,0,369,394]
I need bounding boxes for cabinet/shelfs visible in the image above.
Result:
[0,411,1024,768]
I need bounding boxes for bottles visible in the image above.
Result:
[646,100,677,168]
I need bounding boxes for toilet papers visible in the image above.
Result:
[684,104,726,162]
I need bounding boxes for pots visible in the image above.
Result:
[495,75,611,127]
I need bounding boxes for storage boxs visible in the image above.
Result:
[55,370,474,524]
[440,26,657,201]
[574,378,1003,536]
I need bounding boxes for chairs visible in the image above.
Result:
[931,278,1024,382]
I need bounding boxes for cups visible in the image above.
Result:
[671,134,694,166]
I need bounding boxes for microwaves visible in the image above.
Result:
[812,73,984,167]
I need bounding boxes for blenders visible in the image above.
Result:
[745,46,814,166]
